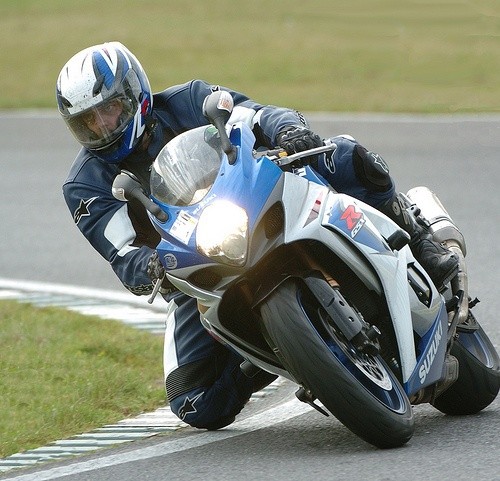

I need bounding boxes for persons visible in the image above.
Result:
[56,42,459,431]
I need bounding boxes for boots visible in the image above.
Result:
[379,194,460,289]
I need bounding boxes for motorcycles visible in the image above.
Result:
[111,89,500,451]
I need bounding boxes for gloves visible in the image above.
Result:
[146,251,178,294]
[277,125,323,170]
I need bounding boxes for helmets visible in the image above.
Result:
[56,41,153,165]
